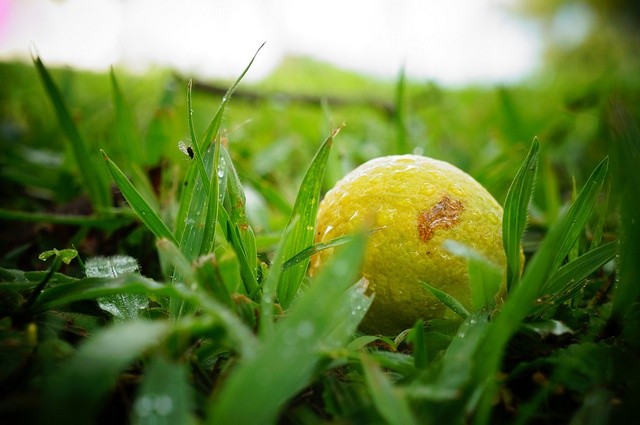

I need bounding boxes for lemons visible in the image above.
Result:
[307,146,526,336]
